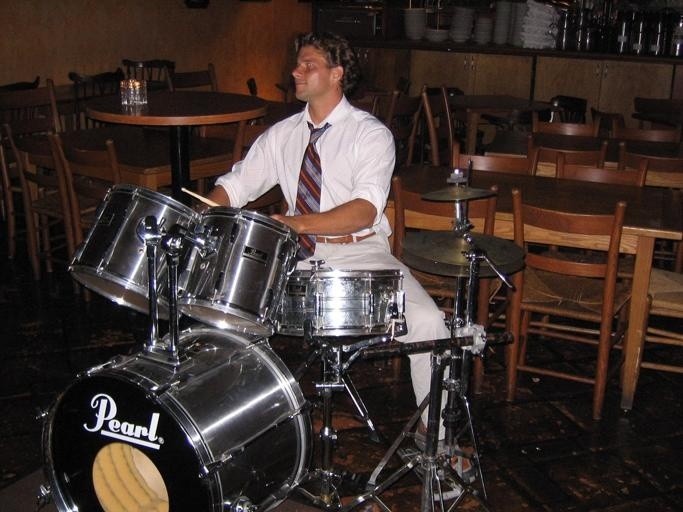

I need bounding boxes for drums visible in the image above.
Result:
[284,268,407,340]
[175,206,298,335]
[68,184,201,321]
[35,325,313,512]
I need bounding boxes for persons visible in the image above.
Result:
[192,29,478,486]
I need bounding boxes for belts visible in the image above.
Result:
[316,232,376,243]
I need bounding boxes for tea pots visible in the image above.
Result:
[545,10,578,52]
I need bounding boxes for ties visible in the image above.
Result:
[293,122,332,260]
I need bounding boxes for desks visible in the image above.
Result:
[381,163,682,412]
[86,91,267,201]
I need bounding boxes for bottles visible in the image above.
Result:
[119,79,147,106]
[575,3,682,59]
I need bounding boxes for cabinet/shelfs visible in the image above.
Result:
[410,51,533,106]
[532,55,673,130]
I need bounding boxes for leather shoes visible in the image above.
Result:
[414,421,472,472]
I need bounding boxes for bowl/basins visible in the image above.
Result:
[403,2,530,46]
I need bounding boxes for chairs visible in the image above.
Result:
[2,75,61,134]
[391,174,502,398]
[0,144,60,274]
[163,62,218,96]
[122,58,175,89]
[5,123,75,286]
[67,67,126,117]
[503,189,635,421]
[361,91,682,189]
[56,131,118,247]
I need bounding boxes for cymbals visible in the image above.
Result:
[400,230,526,267]
[404,253,526,279]
[422,187,496,201]
